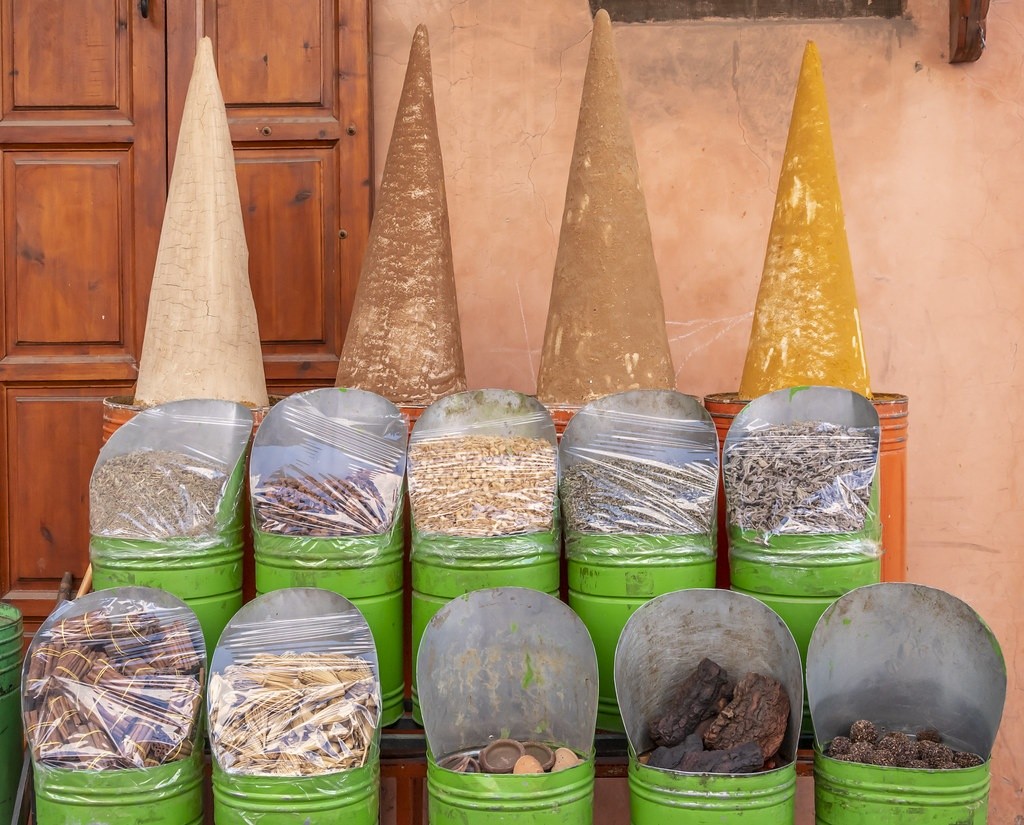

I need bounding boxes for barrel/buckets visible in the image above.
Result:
[627,753,798,823]
[0,600,23,825]
[88,529,247,739]
[425,751,596,825]
[810,746,991,825]
[253,526,887,738]
[27,758,201,824]
[208,757,379,824]
